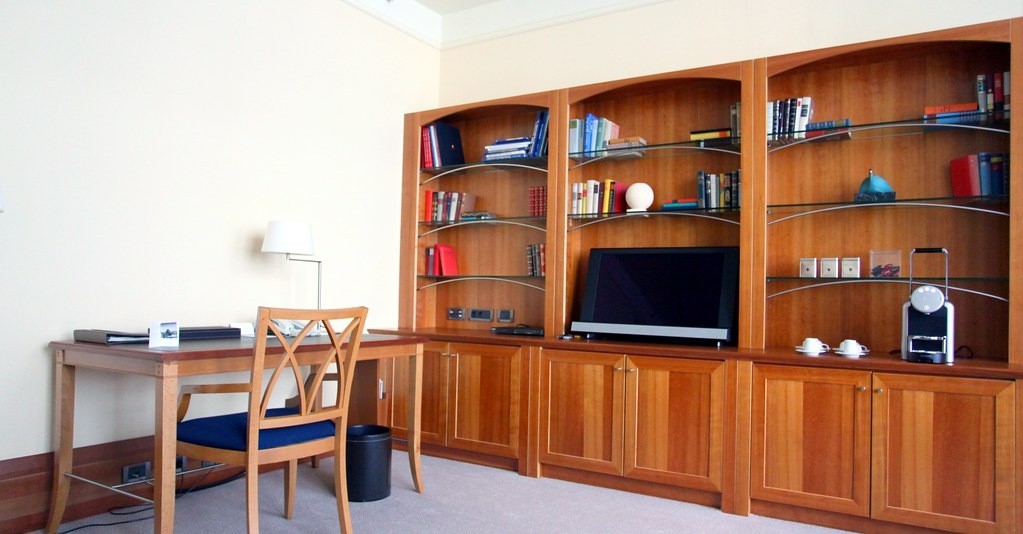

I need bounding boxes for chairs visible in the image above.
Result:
[178,304,368,534]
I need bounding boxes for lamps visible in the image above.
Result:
[262,219,323,337]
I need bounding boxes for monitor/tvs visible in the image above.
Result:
[581,247,740,333]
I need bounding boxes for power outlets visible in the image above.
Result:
[122,455,223,484]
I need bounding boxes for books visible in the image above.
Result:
[527,243,545,277]
[73,329,149,344]
[569,113,647,158]
[923,71,1010,131]
[425,190,496,225]
[424,244,457,276]
[951,152,1010,207]
[496,327,543,335]
[481,109,549,162]
[422,123,465,170]
[571,179,627,218]
[529,186,547,216]
[663,171,740,213]
[689,102,741,149]
[767,96,851,147]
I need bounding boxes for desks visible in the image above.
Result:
[44,326,431,534]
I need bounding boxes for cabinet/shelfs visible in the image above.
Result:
[366,15,1022,533]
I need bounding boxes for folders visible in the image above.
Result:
[72,329,148,346]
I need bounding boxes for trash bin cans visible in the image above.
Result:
[345,423,393,502]
